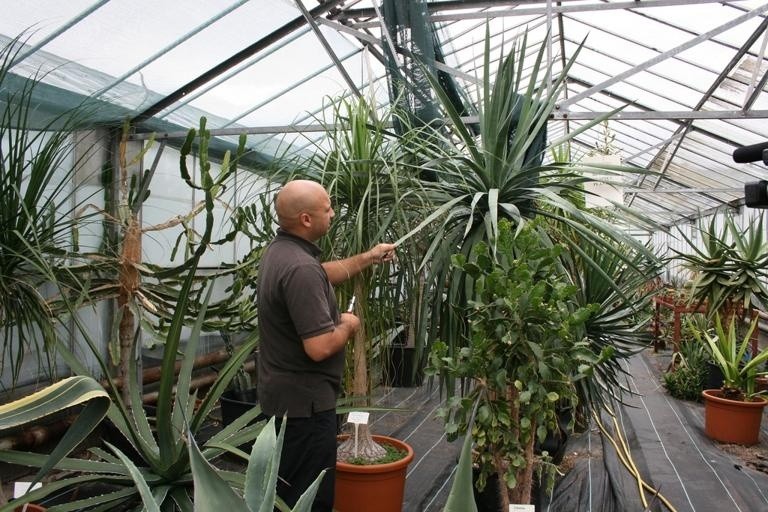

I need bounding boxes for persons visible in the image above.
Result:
[255,179,398,511]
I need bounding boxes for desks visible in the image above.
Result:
[651,296,758,372]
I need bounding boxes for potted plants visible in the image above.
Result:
[686,309,768,446]
[218,44,548,511]
